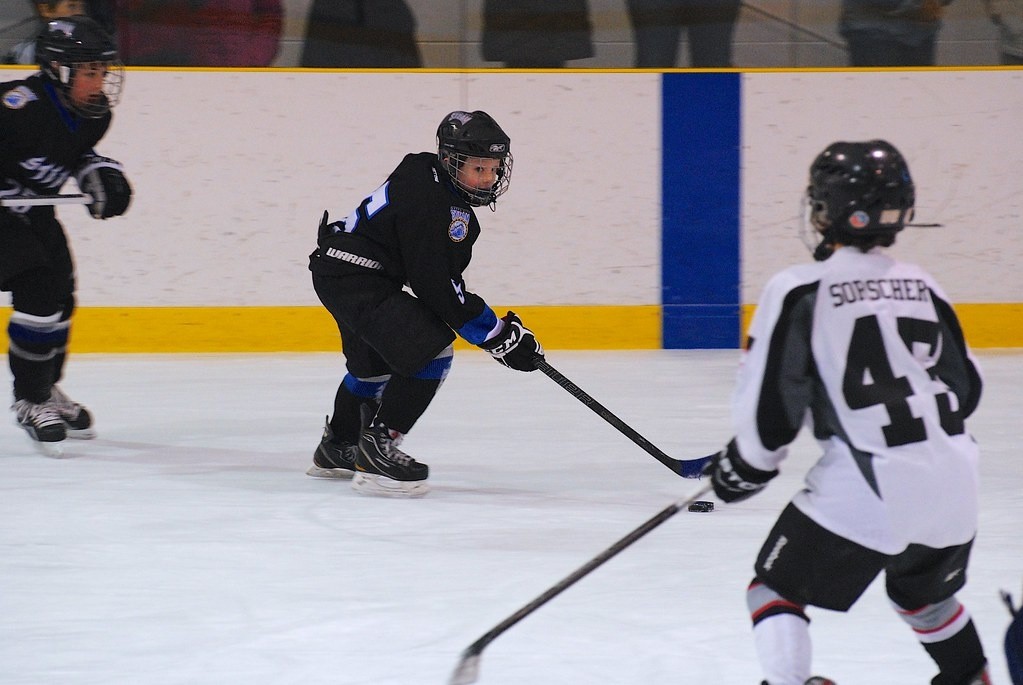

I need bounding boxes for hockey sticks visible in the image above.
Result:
[0,194,93,208]
[537,359,726,480]
[447,481,713,685]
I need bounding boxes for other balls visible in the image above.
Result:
[688,501,714,513]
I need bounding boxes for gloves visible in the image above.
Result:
[482,311,544,371]
[700,449,765,503]
[72,155,132,217]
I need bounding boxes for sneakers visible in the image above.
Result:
[13,398,67,459]
[49,385,94,440]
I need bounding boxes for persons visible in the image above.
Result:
[626,0,741,73]
[116,0,283,67]
[1,0,88,67]
[708,139,991,685]
[480,0,594,69]
[0,14,134,443]
[310,107,536,494]
[301,0,422,68]
[838,0,953,67]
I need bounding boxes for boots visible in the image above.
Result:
[351,402,430,499]
[307,415,358,478]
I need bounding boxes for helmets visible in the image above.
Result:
[36,11,125,118]
[435,110,513,208]
[800,139,916,259]
